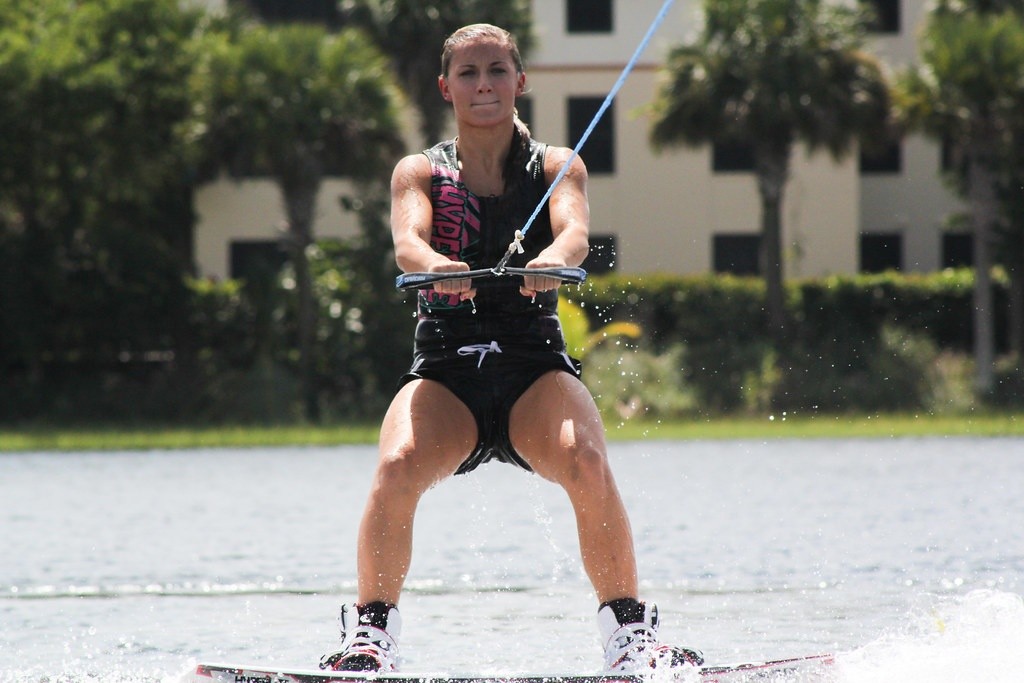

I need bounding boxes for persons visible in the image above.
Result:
[324,23,703,672]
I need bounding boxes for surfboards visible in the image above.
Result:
[196,653,837,683]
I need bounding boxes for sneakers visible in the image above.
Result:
[595,598,706,673]
[318,598,404,673]
